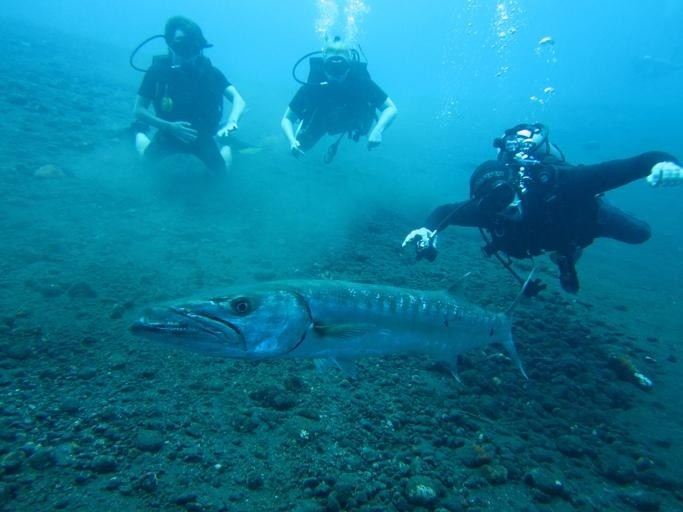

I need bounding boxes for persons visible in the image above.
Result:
[128,16,245,181]
[281,39,396,160]
[402,150,682,295]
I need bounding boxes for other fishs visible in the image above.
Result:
[128,265,536,390]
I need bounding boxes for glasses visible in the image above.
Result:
[170,40,203,57]
[325,63,346,79]
[474,169,514,219]
